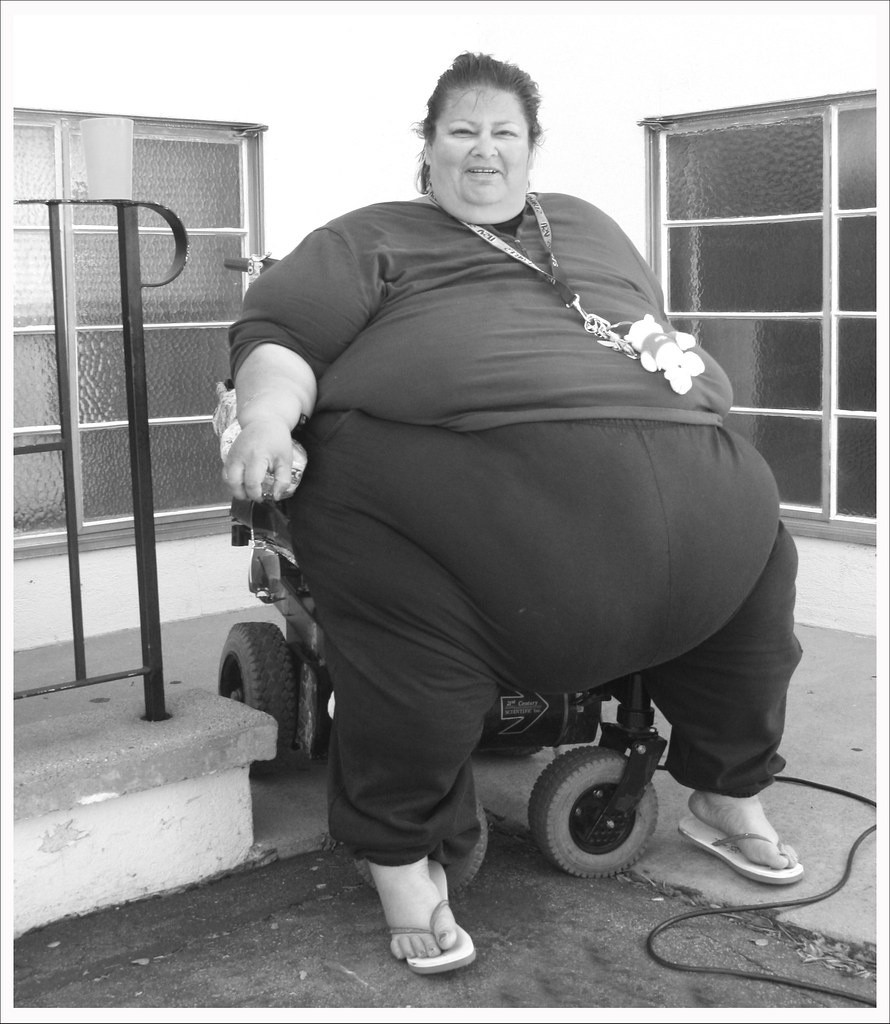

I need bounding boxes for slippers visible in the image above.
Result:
[678,813,804,884]
[388,860,476,975]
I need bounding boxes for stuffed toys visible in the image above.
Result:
[624,313,706,395]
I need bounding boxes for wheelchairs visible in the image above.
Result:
[217,253,669,890]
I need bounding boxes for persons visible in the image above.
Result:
[223,53,805,973]
